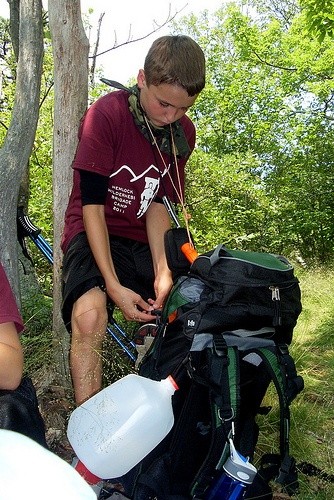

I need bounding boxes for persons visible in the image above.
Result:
[1,261,27,393]
[57,35,205,500]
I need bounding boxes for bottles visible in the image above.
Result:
[66,374,180,479]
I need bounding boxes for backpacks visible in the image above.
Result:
[98,245,303,499]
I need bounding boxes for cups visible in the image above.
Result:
[204,438,257,500]
